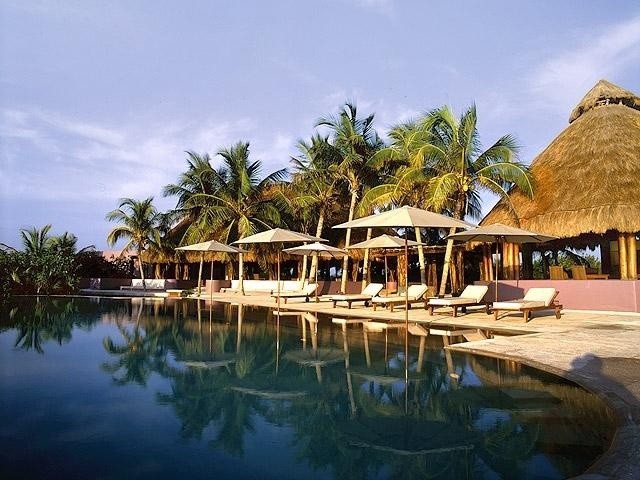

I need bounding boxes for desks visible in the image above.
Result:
[586,274,609,280]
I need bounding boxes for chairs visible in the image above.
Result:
[429,323,489,342]
[571,266,587,280]
[489,287,562,323]
[363,320,428,337]
[273,309,319,323]
[549,266,564,280]
[371,285,429,312]
[271,284,319,304]
[329,283,383,309]
[331,315,371,323]
[427,284,493,317]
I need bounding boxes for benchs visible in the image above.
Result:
[166,289,188,297]
[219,280,309,294]
[120,278,166,291]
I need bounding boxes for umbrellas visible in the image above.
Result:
[173,240,252,301]
[225,307,312,400]
[231,227,330,307]
[332,320,486,461]
[284,312,350,368]
[339,328,428,386]
[174,301,235,369]
[343,234,427,297]
[331,205,482,319]
[444,222,560,302]
[440,358,563,416]
[282,242,347,301]
